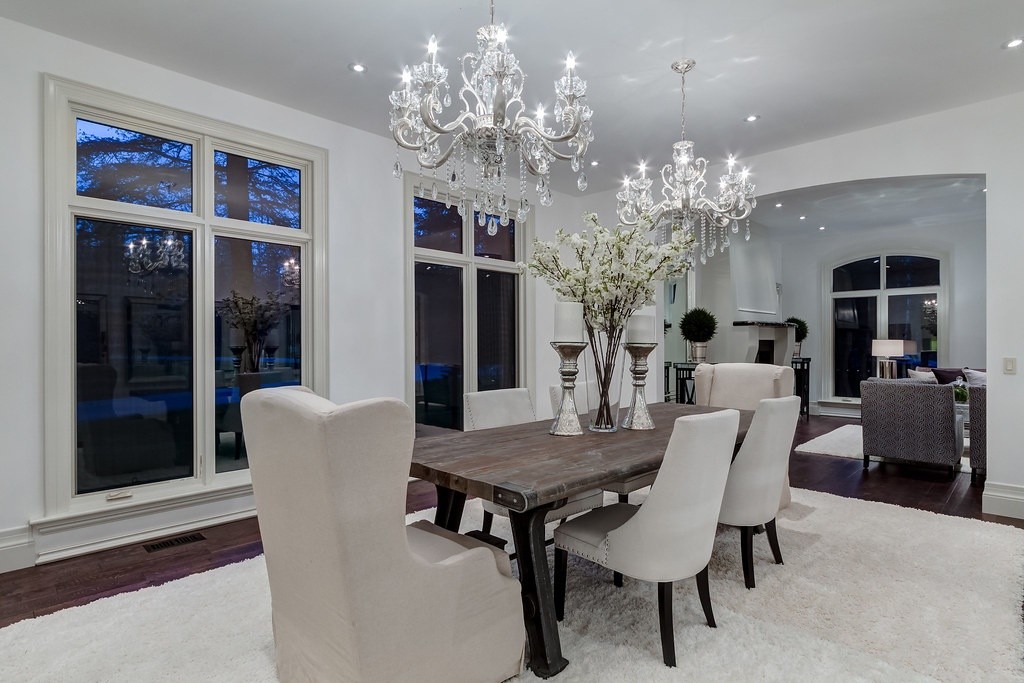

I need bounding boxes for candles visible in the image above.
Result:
[554,302,583,343]
[627,315,655,344]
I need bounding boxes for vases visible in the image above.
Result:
[583,326,628,433]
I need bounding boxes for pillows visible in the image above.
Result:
[908,369,938,383]
[963,369,987,386]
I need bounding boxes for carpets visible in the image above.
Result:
[795,424,972,473]
[0,484,1024,683]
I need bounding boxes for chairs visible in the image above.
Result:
[554,408,740,668]
[240,386,526,682]
[861,381,964,480]
[719,396,802,588]
[462,388,603,562]
[969,386,987,482]
[549,382,659,503]
[695,363,795,510]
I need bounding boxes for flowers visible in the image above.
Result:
[516,212,694,326]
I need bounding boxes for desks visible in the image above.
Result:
[409,403,767,678]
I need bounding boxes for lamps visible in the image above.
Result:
[389,1,595,234]
[616,58,756,265]
[872,340,903,378]
